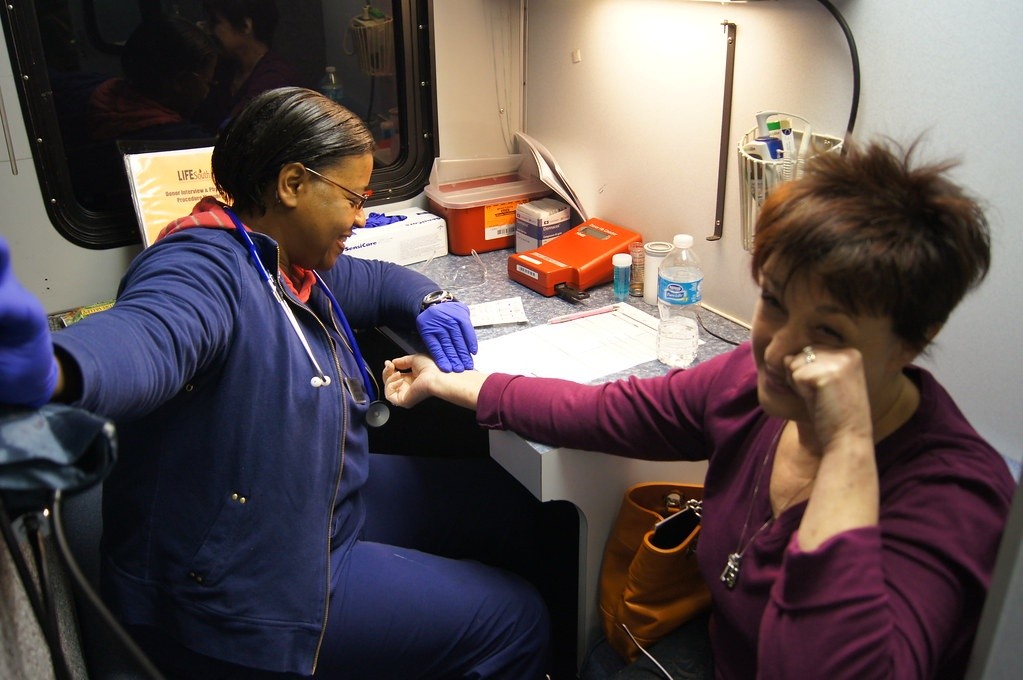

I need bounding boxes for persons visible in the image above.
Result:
[0,86,549,680]
[60,16,218,224]
[382,136,1017,680]
[202,0,315,137]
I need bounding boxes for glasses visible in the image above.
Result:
[277,161,369,210]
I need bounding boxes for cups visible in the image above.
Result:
[643,242,674,307]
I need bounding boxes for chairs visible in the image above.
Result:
[51,481,164,680]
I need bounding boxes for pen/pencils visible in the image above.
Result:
[547,305,620,325]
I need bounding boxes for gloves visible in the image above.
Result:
[0,237,57,410]
[416,301,478,373]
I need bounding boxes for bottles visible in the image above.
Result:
[656,234,703,369]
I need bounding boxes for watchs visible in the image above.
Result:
[419,290,460,314]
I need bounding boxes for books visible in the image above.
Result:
[515,130,589,222]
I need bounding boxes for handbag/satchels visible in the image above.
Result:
[599,482,720,665]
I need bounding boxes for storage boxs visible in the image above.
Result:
[426,154,549,255]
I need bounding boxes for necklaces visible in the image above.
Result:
[719,378,907,589]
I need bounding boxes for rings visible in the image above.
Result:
[803,346,815,362]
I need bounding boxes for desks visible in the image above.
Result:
[377,252,753,678]
